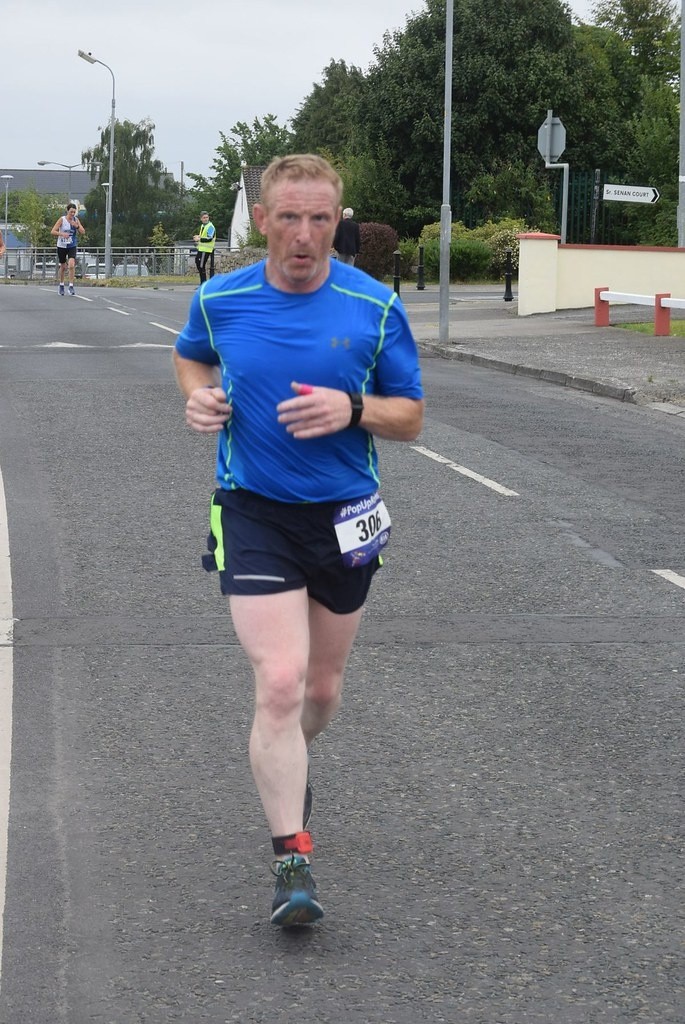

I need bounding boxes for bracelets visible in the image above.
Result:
[76,226,79,229]
[346,392,364,427]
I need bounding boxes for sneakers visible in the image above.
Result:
[67,285,76,297]
[268,850,325,925]
[57,284,65,296]
[302,765,315,831]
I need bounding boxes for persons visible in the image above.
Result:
[193,211,216,290]
[333,207,360,267]
[51,204,86,295]
[0,230,5,256]
[175,156,425,924]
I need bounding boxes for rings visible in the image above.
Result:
[298,384,313,395]
